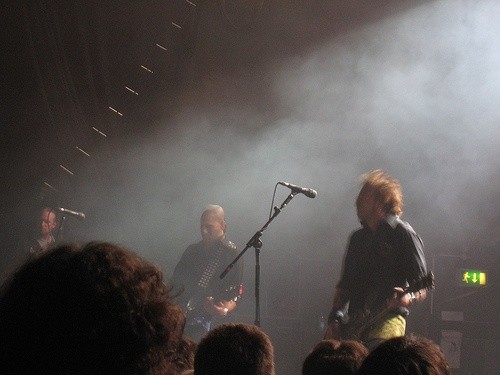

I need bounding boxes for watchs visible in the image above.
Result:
[410,291,416,307]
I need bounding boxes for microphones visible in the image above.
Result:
[59,207,85,219]
[279,182,317,198]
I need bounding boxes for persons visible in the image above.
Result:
[167,204,246,345]
[25,206,58,264]
[0,239,452,375]
[324,172,429,354]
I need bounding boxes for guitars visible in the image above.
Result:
[178,282,243,312]
[324,272,435,344]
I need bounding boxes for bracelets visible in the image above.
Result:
[223,307,228,318]
